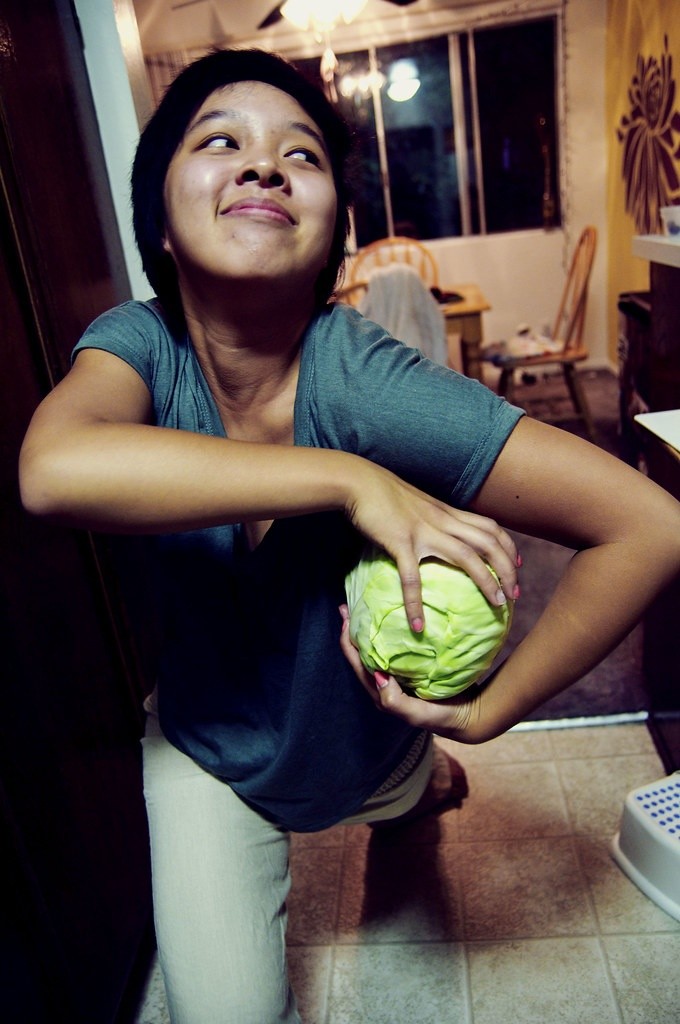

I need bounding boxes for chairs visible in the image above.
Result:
[332,235,439,311]
[481,225,599,440]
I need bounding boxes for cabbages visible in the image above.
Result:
[347,536,514,700]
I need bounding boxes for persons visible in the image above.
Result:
[18,49,680,1023]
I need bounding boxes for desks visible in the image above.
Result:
[429,281,493,386]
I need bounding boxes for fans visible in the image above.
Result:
[171,0,417,31]
